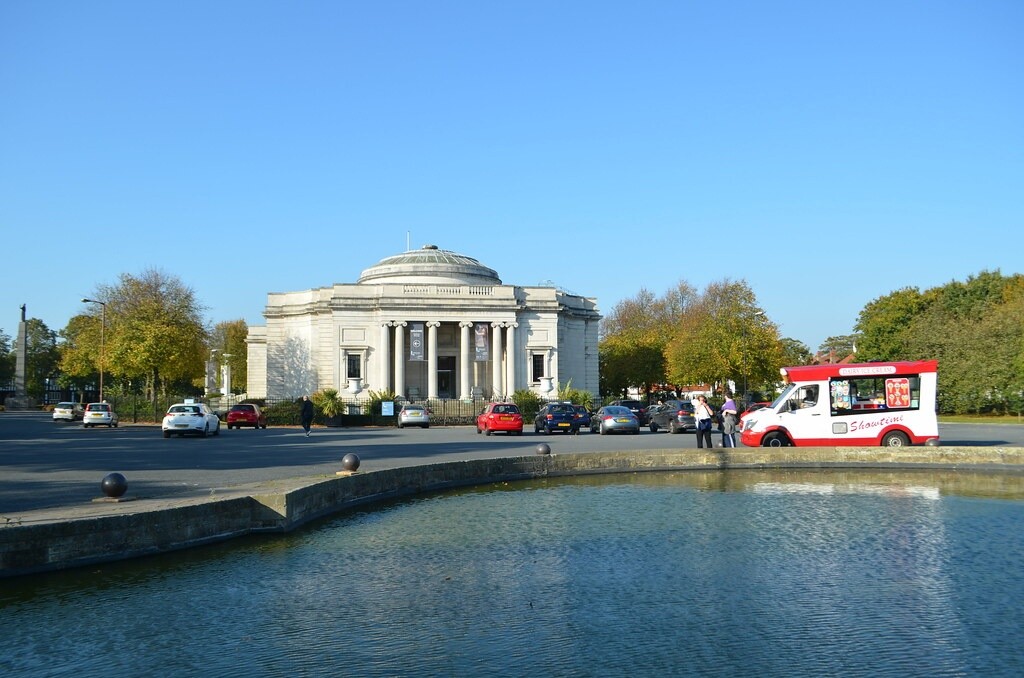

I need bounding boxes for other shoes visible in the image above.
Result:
[307,431,311,435]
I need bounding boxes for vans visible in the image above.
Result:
[738,360,941,447]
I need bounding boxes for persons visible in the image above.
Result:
[694,389,814,448]
[300,395,312,438]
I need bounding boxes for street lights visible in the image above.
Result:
[81,298,106,410]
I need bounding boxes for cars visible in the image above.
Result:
[571,405,590,427]
[161,402,221,438]
[396,404,430,428]
[643,404,660,424]
[587,406,641,435]
[740,401,775,421]
[53,402,85,422]
[476,402,523,435]
[608,399,646,427]
[647,400,716,434]
[82,402,118,428]
[227,404,266,430]
[534,402,580,435]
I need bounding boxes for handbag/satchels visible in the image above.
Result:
[700,420,712,430]
